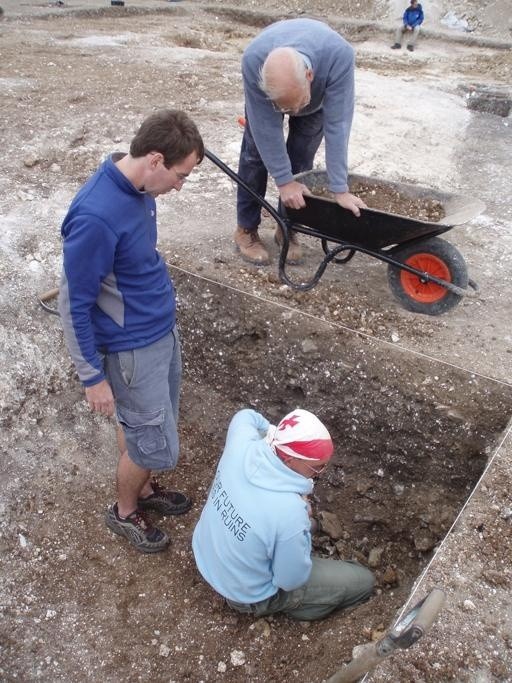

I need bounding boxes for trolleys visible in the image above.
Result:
[200,147,485,314]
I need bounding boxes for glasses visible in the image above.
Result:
[269,94,310,114]
[298,459,327,476]
[172,166,188,184]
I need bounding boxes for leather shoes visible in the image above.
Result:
[274,223,303,265]
[234,224,271,266]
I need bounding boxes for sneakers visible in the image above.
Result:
[104,501,170,554]
[138,483,192,514]
[391,43,401,49]
[407,44,413,52]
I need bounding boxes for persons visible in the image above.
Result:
[191,409,375,622]
[390,1,423,52]
[232,18,369,266]
[58,108,204,554]
